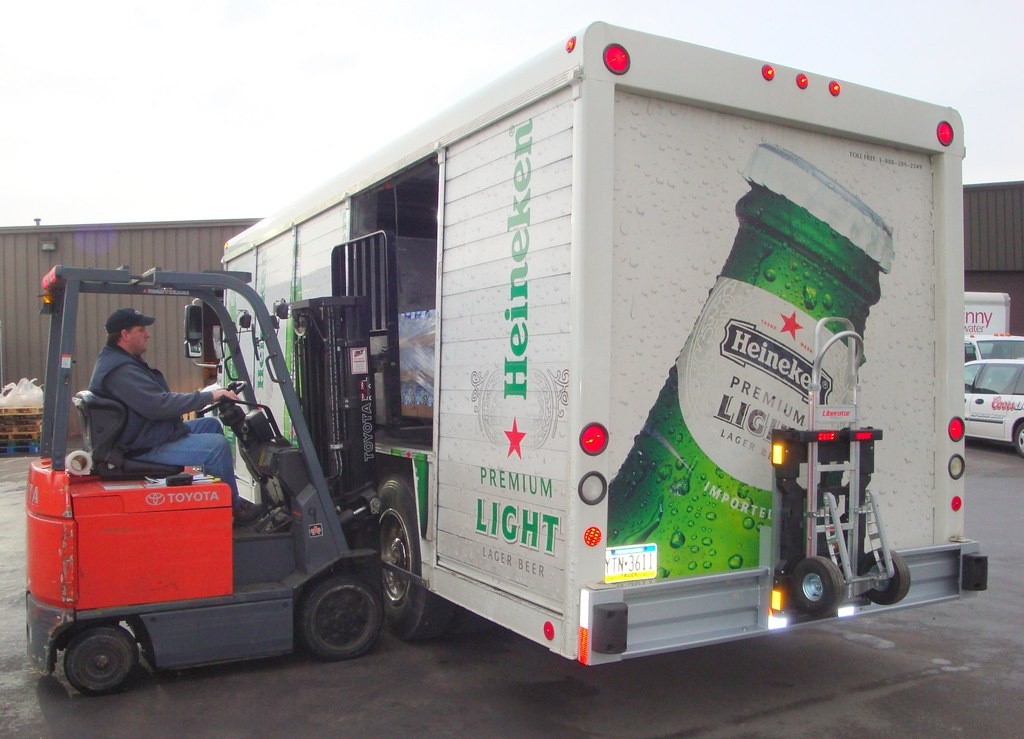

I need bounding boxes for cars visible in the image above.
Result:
[964,357,1024,459]
[965,331,1023,362]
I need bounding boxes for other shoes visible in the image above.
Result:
[233,496,268,526]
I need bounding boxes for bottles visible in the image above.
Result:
[608,143,896,583]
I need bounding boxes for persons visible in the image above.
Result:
[88,307,269,529]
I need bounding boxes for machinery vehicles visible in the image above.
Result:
[21,231,432,697]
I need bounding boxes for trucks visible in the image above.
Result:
[180,20,969,666]
[964,292,1011,335]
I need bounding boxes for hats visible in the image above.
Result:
[105,308,155,332]
